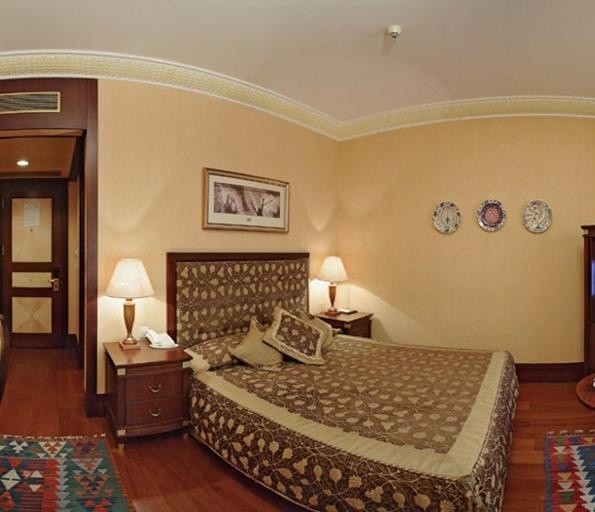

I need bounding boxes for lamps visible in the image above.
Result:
[104,259,155,349]
[314,254,348,316]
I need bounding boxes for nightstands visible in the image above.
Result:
[105,339,191,440]
[316,309,374,339]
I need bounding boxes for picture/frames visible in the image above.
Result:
[203,166,291,234]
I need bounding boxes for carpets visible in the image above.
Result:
[541,428,594,512]
[1,432,135,512]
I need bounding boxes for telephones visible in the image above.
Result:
[145,328,178,349]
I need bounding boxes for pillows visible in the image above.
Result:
[229,315,284,366]
[183,330,249,371]
[261,305,328,365]
[298,307,336,346]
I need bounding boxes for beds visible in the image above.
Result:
[165,251,523,511]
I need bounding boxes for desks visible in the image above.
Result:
[575,372,595,409]
